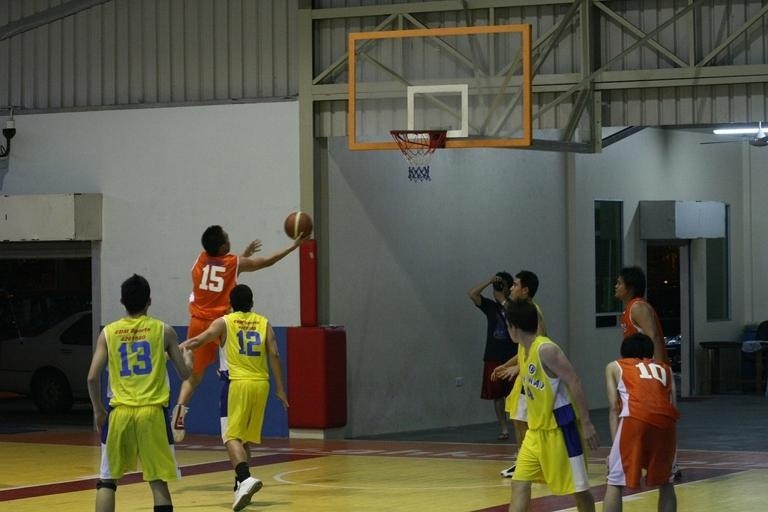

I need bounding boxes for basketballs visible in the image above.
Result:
[285,212,313,240]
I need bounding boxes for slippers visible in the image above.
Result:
[499,432,509,439]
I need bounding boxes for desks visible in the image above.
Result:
[699,341,767,394]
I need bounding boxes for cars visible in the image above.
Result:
[0,311,93,410]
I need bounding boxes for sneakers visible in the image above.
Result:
[232,476,262,511]
[171,404,189,443]
[501,463,516,477]
[672,464,678,474]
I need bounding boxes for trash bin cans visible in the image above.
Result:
[699,341,742,395]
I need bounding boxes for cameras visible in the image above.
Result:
[491,281,504,291]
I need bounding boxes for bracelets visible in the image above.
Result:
[500,298,508,306]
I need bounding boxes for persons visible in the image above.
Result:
[171,225,311,443]
[468,272,517,439]
[614,267,683,479]
[505,298,602,512]
[491,271,543,479]
[164,285,290,512]
[87,274,194,512]
[603,335,676,512]
